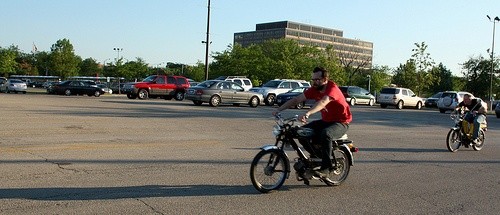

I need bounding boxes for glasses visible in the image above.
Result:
[310,78,326,82]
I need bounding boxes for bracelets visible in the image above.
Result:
[305,115,309,118]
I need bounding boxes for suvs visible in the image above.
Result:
[437,91,476,113]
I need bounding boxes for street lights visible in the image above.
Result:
[367,74,371,91]
[487,15,500,101]
[113,48,123,94]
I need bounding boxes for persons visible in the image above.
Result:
[272,66,353,180]
[454,94,486,144]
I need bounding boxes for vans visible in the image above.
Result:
[339,86,376,106]
[216,76,252,90]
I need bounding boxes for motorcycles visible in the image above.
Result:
[446,106,487,153]
[250,113,359,192]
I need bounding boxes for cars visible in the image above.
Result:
[425,92,443,108]
[97,84,112,94]
[185,80,265,108]
[30,81,51,88]
[121,75,190,100]
[54,81,105,97]
[47,82,62,94]
[377,87,424,110]
[0,77,27,93]
[276,86,316,109]
[249,80,305,106]
[494,101,500,119]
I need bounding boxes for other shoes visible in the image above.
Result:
[473,138,477,144]
[315,168,328,178]
